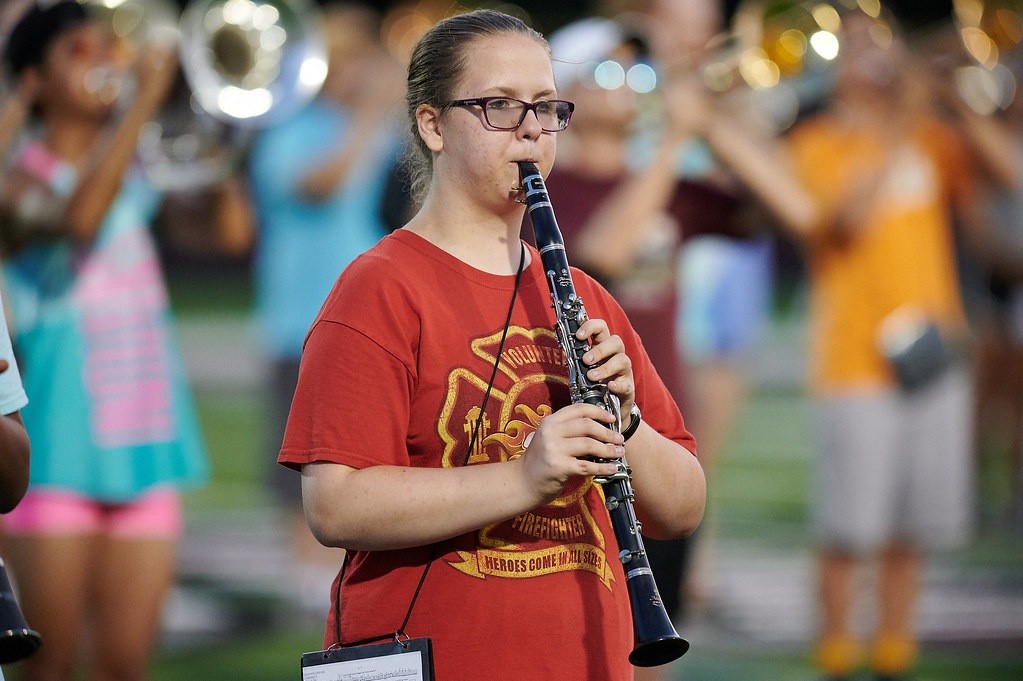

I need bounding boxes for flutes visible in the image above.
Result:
[510,161,690,669]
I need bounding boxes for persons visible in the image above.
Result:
[0,0,404,681]
[278,9,705,681]
[519,0,1023,681]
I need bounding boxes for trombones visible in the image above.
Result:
[84,0,333,192]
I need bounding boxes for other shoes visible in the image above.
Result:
[819,626,855,678]
[870,632,915,676]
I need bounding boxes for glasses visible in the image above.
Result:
[433,96,575,133]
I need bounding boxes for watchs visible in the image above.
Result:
[621,403,642,442]
